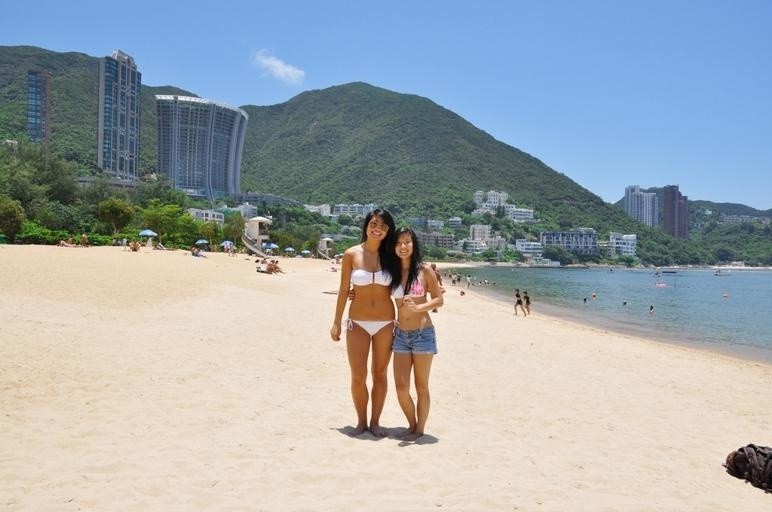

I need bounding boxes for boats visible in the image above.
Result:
[713,270,732,276]
[661,270,676,273]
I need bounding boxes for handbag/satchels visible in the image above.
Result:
[723,443,772,493]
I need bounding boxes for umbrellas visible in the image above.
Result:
[195,239,209,245]
[139,230,158,246]
[220,240,233,246]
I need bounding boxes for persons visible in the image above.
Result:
[57,240,81,248]
[330,208,397,438]
[113,237,116,246]
[331,260,339,272]
[66,236,74,244]
[224,242,228,253]
[522,290,530,315]
[194,247,207,258]
[192,245,196,255]
[336,256,343,264]
[205,244,210,251]
[228,242,238,257]
[80,232,90,247]
[266,259,284,274]
[129,238,141,253]
[348,227,444,442]
[648,304,654,313]
[117,238,122,246]
[513,288,526,316]
[123,237,128,246]
[444,269,496,289]
[429,262,443,313]
